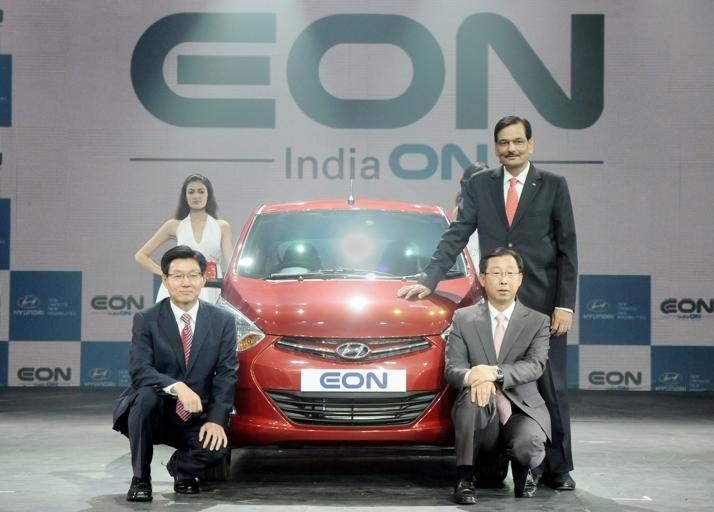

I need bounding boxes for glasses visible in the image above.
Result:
[166,271,204,280]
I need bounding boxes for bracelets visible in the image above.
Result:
[494,365,503,383]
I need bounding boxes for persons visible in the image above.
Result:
[133,173,233,304]
[110,244,240,503]
[439,249,550,507]
[394,115,579,493]
[449,160,489,281]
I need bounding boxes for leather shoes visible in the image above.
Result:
[129,476,154,503]
[518,469,536,498]
[543,473,576,490]
[455,477,479,504]
[174,477,200,495]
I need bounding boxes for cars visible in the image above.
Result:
[204,198,483,475]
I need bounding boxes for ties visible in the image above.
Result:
[176,313,194,422]
[505,178,520,230]
[493,313,512,426]
